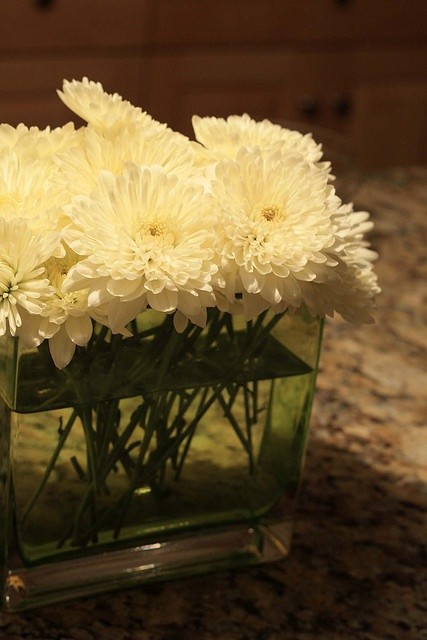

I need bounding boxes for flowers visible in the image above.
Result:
[1,76,382,549]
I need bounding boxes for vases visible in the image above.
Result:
[0,314,327,613]
